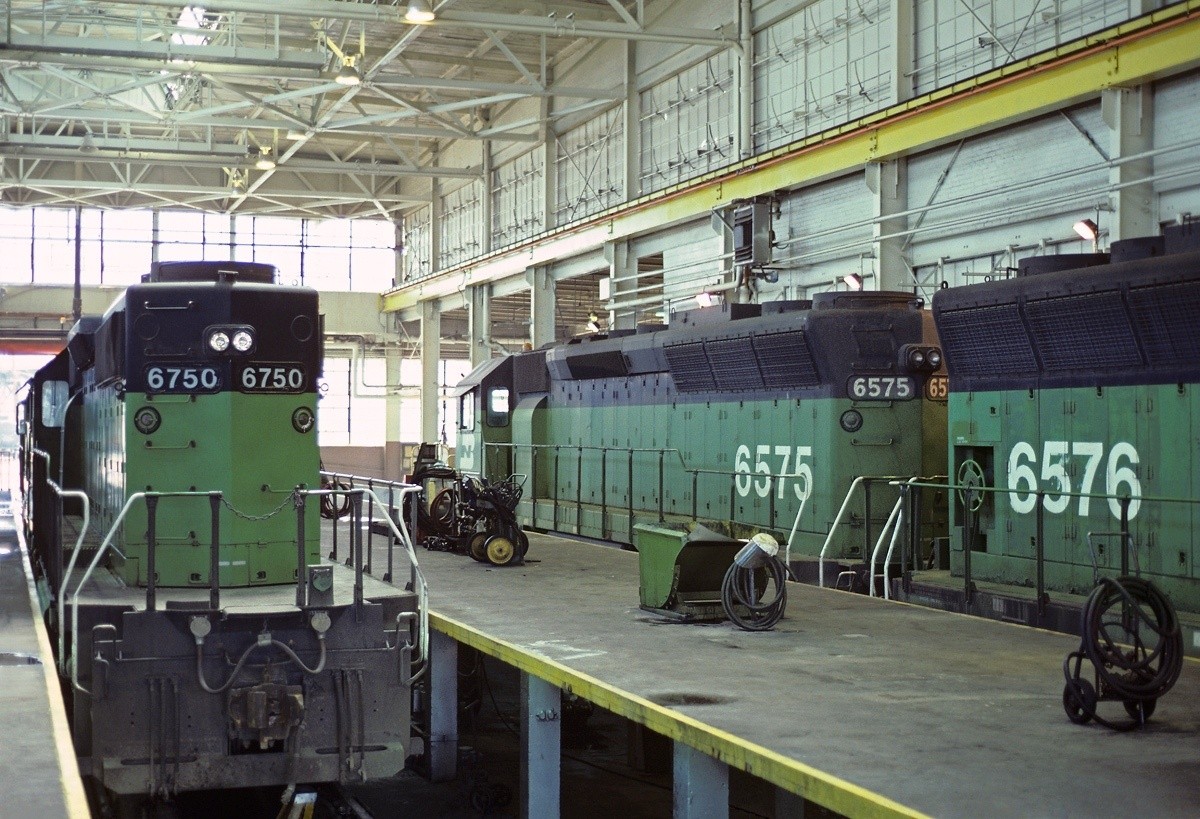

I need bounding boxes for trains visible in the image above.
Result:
[443,215,1200,651]
[22,252,408,795]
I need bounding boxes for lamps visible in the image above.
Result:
[256,154,275,169]
[587,274,597,322]
[1072,219,1097,239]
[844,273,862,290]
[335,67,361,86]
[588,321,599,331]
[230,186,245,199]
[405,0,434,21]
